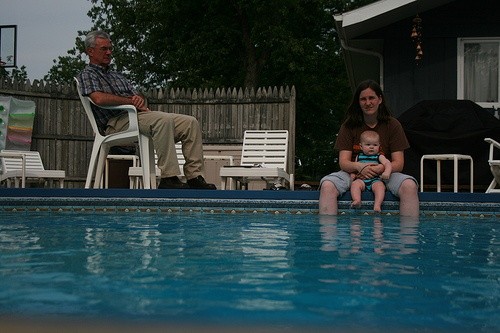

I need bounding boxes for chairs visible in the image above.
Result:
[0,150,66,190]
[220,131,291,194]
[484,138,500,195]
[72,76,185,190]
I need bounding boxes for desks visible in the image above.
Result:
[420,154,473,194]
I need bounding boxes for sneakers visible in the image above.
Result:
[187,175,216,189]
[158,176,189,188]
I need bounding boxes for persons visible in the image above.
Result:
[351,131,392,212]
[319,79,419,215]
[79,30,217,190]
